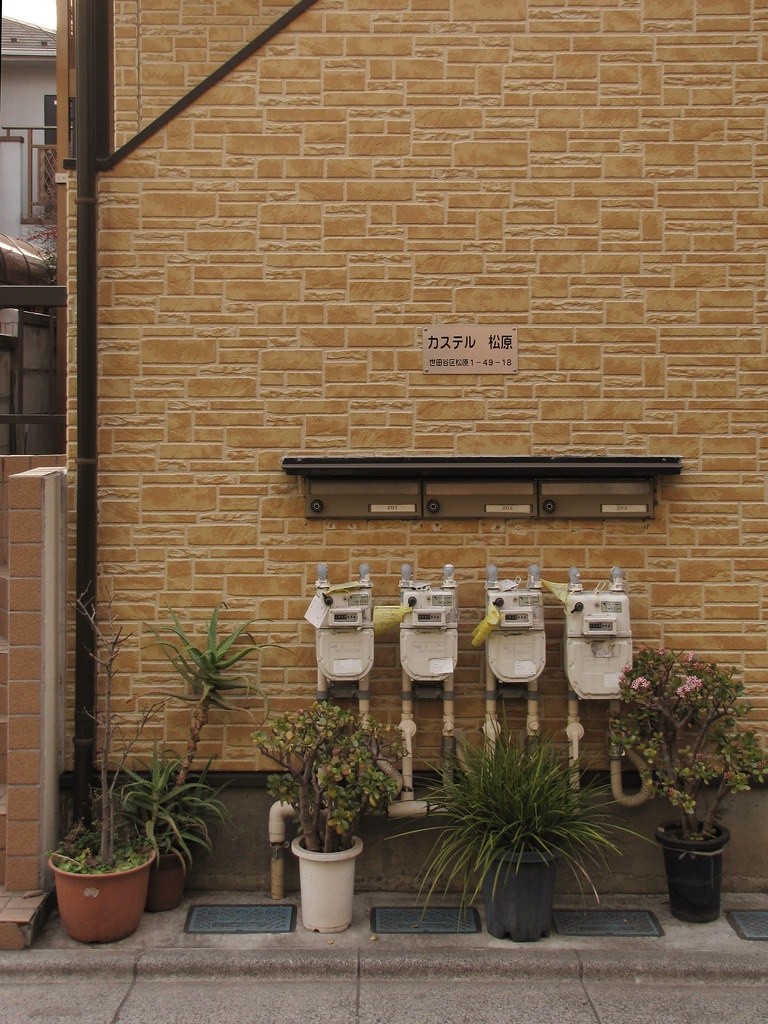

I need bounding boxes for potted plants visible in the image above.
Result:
[252,700,411,933]
[113,595,299,913]
[383,699,662,942]
[41,579,156,944]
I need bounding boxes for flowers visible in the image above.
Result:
[607,642,767,836]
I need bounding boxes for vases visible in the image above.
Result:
[656,820,732,923]
